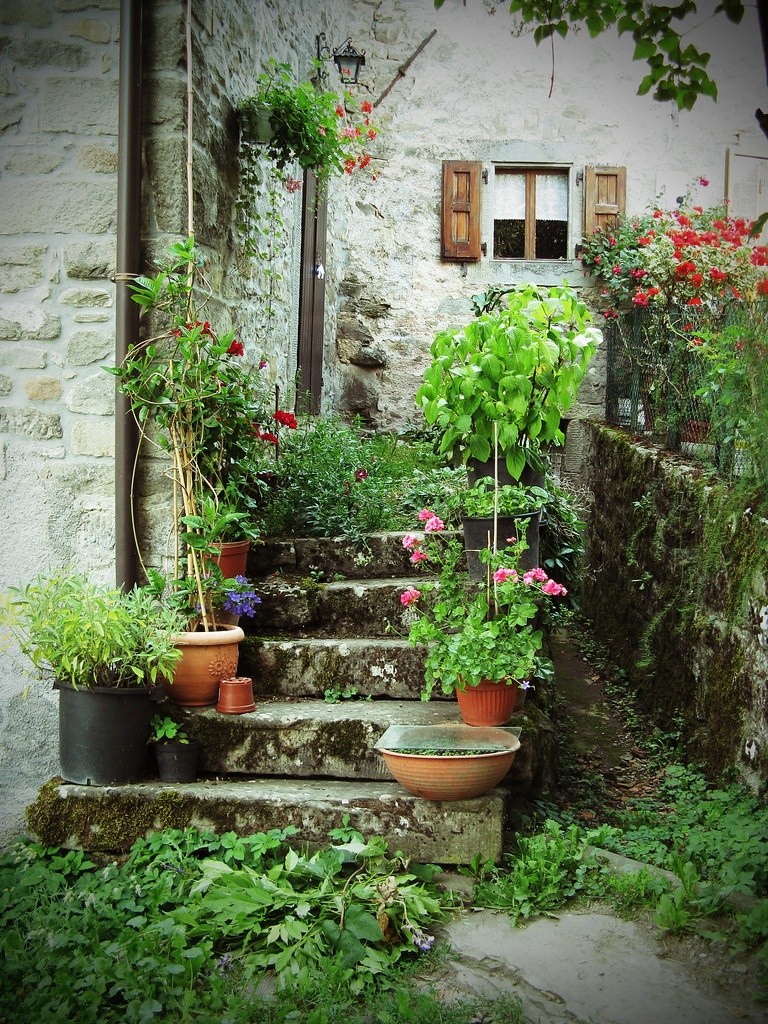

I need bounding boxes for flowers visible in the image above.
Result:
[174,321,298,477]
[153,561,262,631]
[400,509,567,633]
[235,51,380,195]
[576,175,768,349]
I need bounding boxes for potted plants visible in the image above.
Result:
[0,559,184,786]
[378,724,521,802]
[417,621,555,726]
[444,476,556,586]
[416,278,605,489]
[145,715,200,783]
[194,497,264,580]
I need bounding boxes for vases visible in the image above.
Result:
[214,468,274,508]
[467,592,545,633]
[239,104,274,146]
[611,366,716,462]
[155,622,245,707]
[216,676,256,714]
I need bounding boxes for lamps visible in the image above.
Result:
[312,32,365,84]
